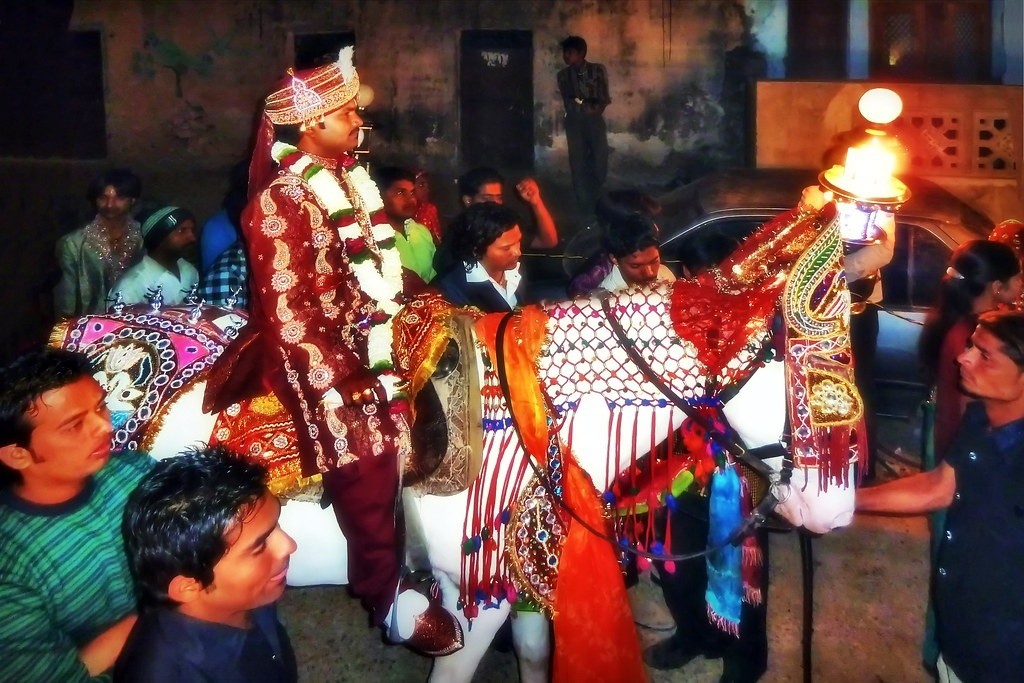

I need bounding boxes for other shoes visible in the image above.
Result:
[644,617,723,669]
[381,589,466,657]
[719,605,767,683]
[627,587,675,630]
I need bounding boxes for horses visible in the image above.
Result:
[46,208,857,683]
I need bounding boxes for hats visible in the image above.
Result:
[141,206,190,249]
[263,46,360,132]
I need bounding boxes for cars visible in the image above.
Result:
[523,167,998,420]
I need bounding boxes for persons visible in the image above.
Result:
[557,35,609,211]
[245,45,465,658]
[0,161,1023,683]
